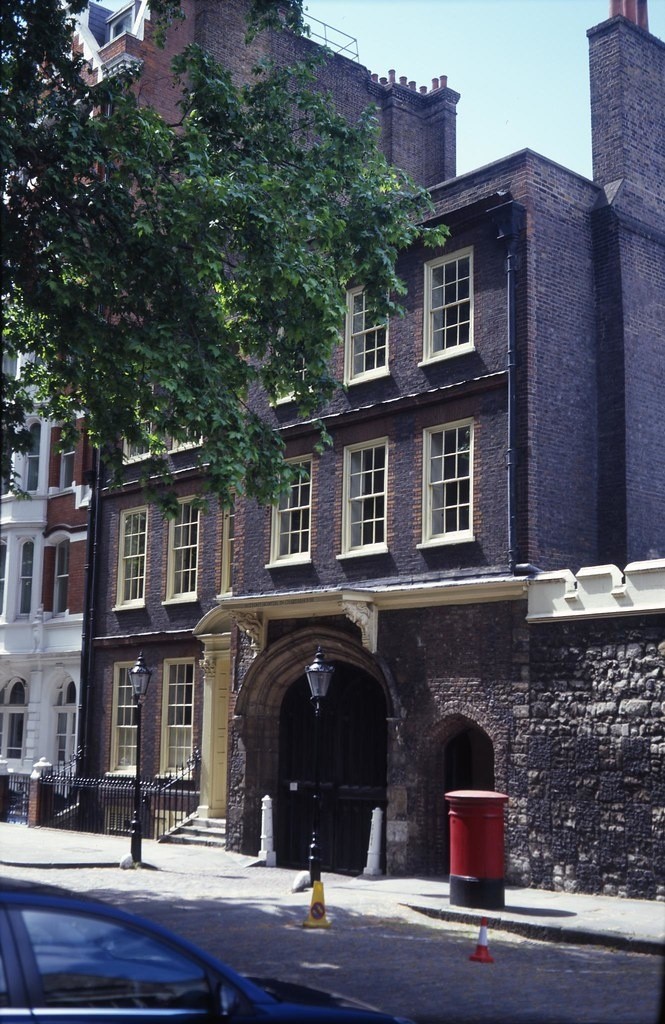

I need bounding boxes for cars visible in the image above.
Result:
[0,877,417,1024]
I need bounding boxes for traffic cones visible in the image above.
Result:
[302,881,333,929]
[467,917,496,965]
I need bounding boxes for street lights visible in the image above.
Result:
[290,644,335,894]
[123,651,155,870]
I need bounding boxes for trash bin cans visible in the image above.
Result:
[442,789,509,911]
